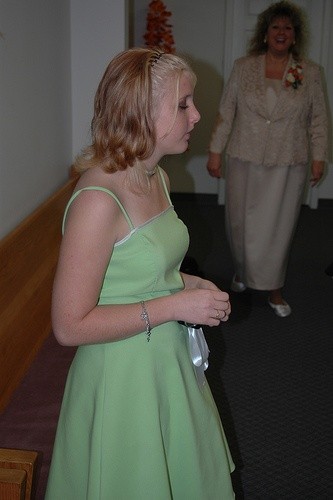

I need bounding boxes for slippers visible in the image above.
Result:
[266,294,290,317]
[231,274,246,292]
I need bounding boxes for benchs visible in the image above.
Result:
[0,163,85,499]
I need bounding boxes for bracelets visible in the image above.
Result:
[139,300,151,342]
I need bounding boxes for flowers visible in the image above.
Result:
[285,64,304,87]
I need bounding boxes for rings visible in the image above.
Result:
[216,310,220,317]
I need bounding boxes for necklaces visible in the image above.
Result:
[145,167,158,178]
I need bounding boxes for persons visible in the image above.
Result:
[41,48,235,500]
[206,0,328,317]
